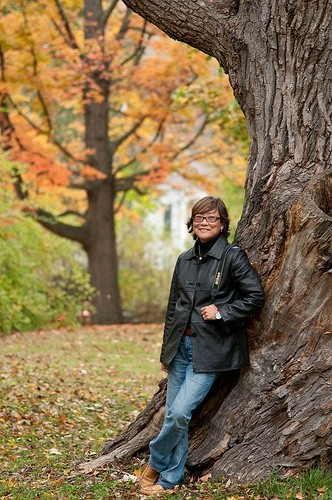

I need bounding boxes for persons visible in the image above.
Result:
[140,196,266,496]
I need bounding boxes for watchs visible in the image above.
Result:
[215,309,222,319]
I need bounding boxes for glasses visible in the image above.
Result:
[192,215,222,223]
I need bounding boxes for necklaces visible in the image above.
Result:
[198,244,210,260]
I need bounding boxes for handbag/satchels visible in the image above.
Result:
[209,244,246,330]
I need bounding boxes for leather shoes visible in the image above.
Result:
[139,484,176,495]
[141,463,158,488]
